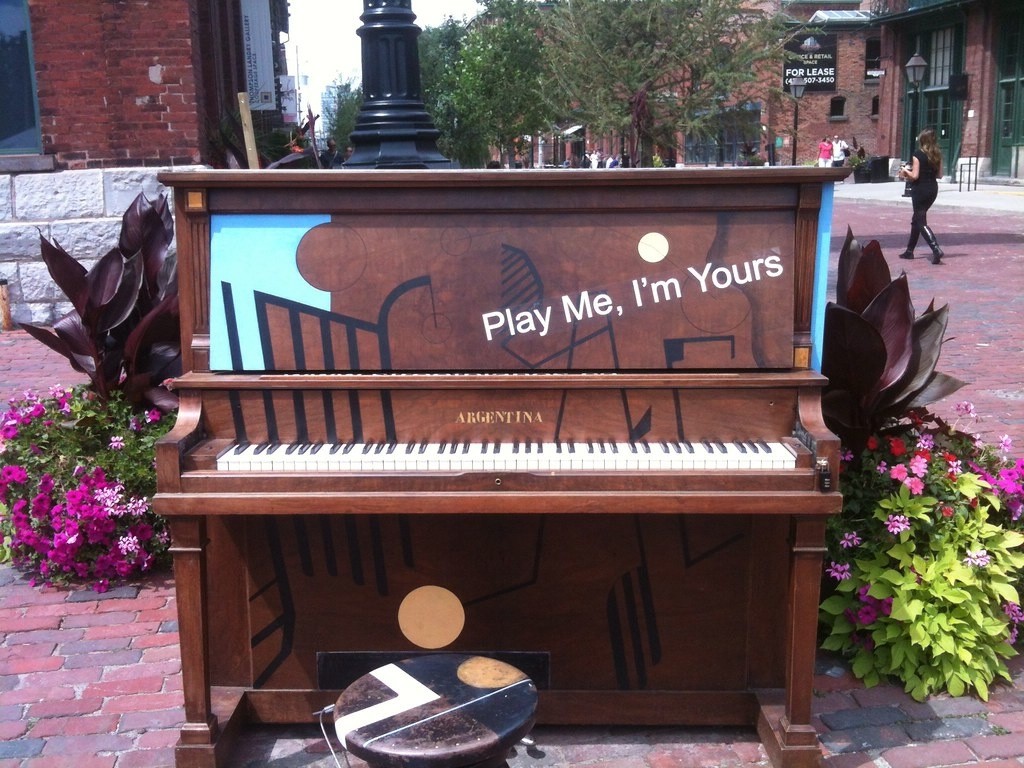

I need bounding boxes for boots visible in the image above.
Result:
[920,226,944,264]
[898,223,920,259]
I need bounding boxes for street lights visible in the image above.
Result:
[788,75,808,166]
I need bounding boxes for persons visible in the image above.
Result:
[320,139,352,169]
[897,128,945,264]
[817,135,848,184]
[520,147,631,169]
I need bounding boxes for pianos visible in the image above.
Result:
[153,170,845,768]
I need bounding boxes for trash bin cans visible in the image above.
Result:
[867,155,890,183]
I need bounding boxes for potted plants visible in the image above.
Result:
[848,156,872,183]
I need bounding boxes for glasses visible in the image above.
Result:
[834,138,839,139]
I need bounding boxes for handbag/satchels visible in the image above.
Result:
[840,140,850,156]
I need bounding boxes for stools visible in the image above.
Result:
[333,654,539,768]
